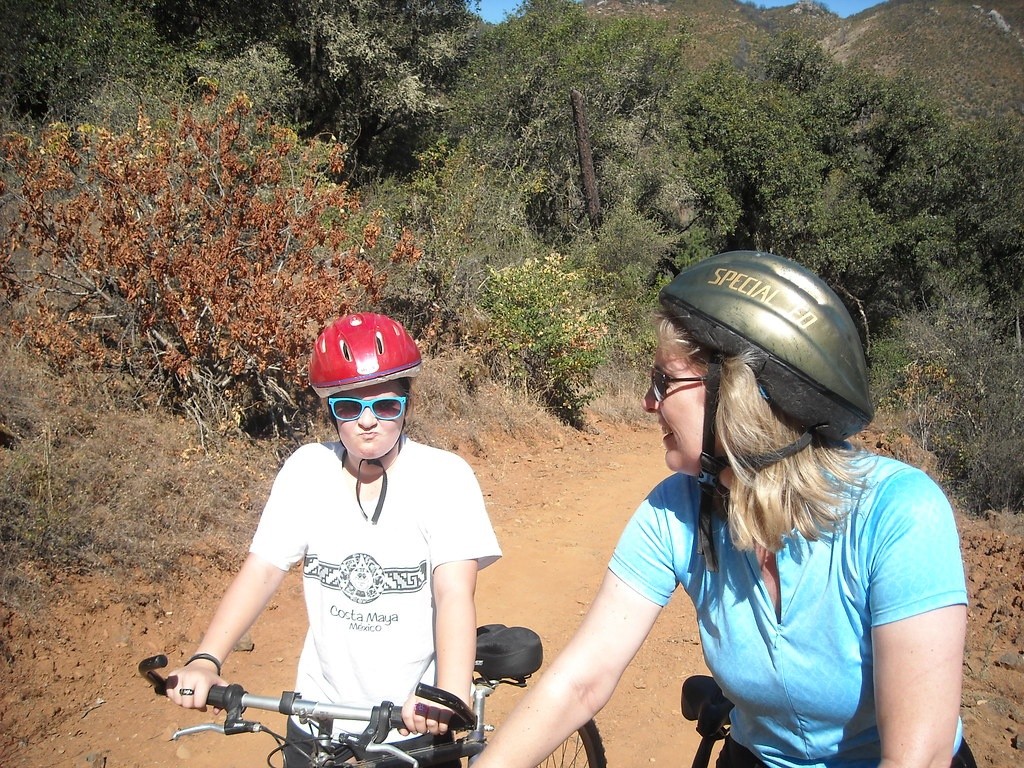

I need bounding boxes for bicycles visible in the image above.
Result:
[137,621,607,766]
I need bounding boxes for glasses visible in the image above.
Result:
[650,367,711,402]
[328,397,406,421]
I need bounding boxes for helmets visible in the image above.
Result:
[310,311,423,399]
[659,251,874,443]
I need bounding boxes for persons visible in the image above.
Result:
[164,308,506,768]
[466,248,984,768]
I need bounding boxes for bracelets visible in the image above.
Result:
[183,653,222,676]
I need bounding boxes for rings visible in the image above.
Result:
[179,689,194,696]
